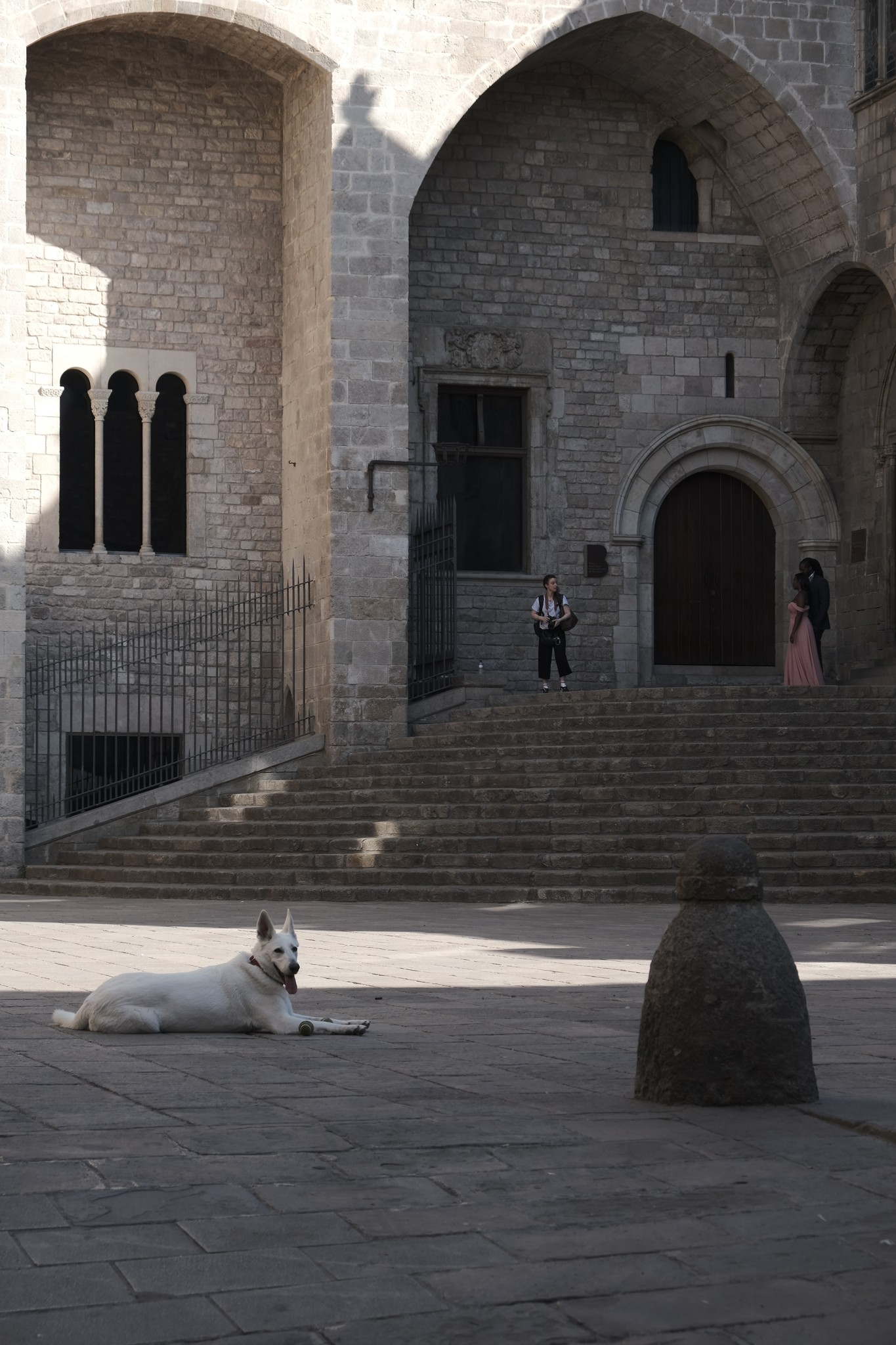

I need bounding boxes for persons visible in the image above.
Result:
[799,557,830,675]
[784,572,825,685]
[531,574,571,692]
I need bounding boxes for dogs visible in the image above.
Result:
[51,909,371,1035]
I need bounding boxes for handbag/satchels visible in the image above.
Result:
[560,594,578,631]
[532,595,544,636]
[541,630,561,646]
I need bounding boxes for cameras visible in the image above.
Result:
[547,615,558,629]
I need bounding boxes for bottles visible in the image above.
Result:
[479,660,484,674]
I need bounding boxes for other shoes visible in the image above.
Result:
[560,685,569,691]
[541,687,549,693]
